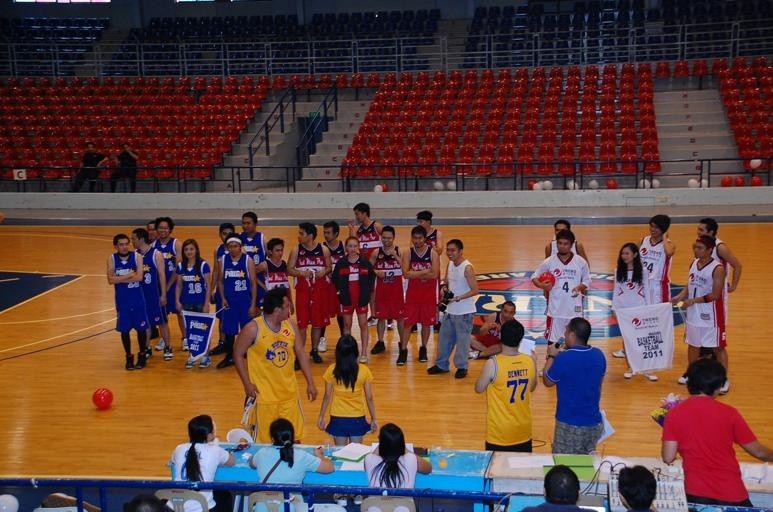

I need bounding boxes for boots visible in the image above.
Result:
[125,351,147,370]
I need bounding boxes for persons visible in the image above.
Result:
[317,335,378,446]
[542,317,606,454]
[73,142,108,192]
[660,360,773,511]
[163,415,236,512]
[109,143,139,193]
[232,288,318,445]
[364,423,432,490]
[475,319,538,452]
[619,466,658,512]
[249,418,335,503]
[125,494,169,512]
[522,465,597,512]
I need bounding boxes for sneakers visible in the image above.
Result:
[427,365,469,379]
[467,350,480,360]
[208,339,236,368]
[145,337,175,361]
[358,340,386,364]
[294,336,328,372]
[417,345,427,363]
[395,341,409,366]
[677,373,730,396]
[609,348,626,359]
[620,367,661,382]
[182,337,190,351]
[364,316,442,334]
[185,355,213,369]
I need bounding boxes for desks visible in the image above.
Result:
[172,445,772,512]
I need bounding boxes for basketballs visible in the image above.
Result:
[639,179,650,188]
[735,176,745,186]
[751,176,763,186]
[374,184,389,192]
[688,179,699,187]
[651,179,660,188]
[539,272,555,287]
[447,182,457,190]
[589,180,598,189]
[699,179,708,187]
[721,176,733,186]
[527,180,553,190]
[750,159,762,169]
[607,179,617,188]
[566,180,578,190]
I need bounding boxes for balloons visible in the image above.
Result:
[566,159,762,191]
[382,184,388,192]
[373,185,383,192]
[446,181,457,191]
[527,178,554,191]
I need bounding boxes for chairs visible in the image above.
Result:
[0,2,268,187]
[674,61,692,80]
[693,58,711,79]
[715,56,773,175]
[657,61,670,80]
[336,65,663,184]
[720,175,745,187]
[270,0,772,56]
[271,73,380,91]
[750,176,762,187]
